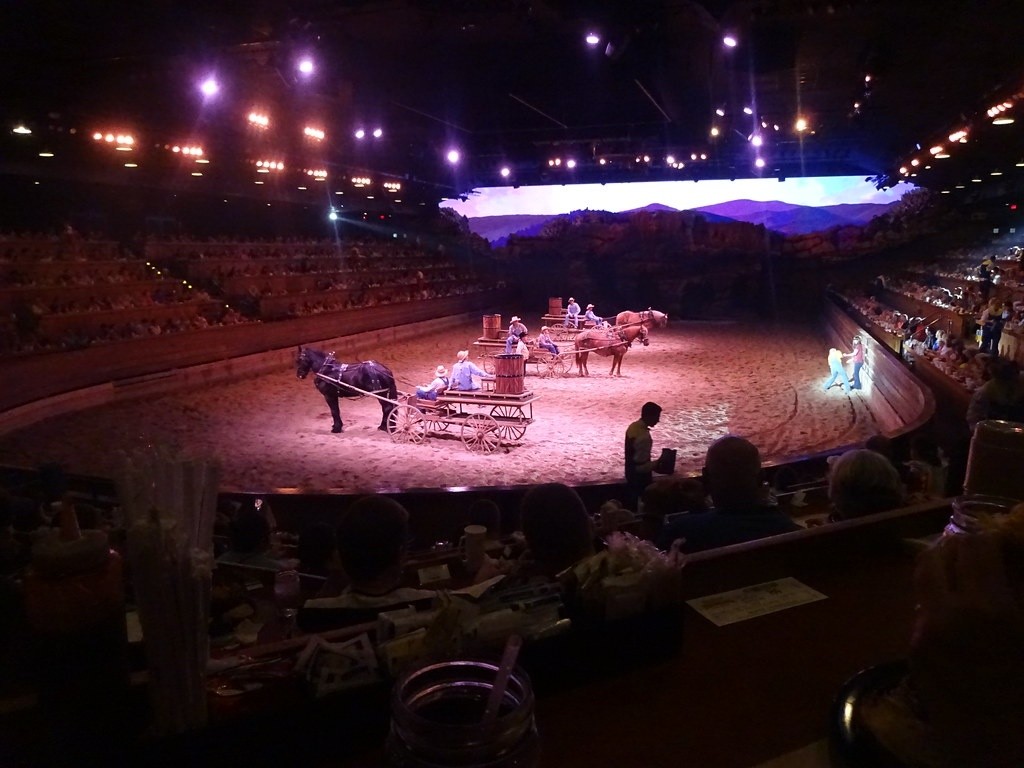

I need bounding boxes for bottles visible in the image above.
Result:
[32,502,127,746]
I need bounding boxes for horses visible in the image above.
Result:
[616,309,668,343]
[294,344,398,434]
[574,325,649,378]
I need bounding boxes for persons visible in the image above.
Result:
[0,191,1024,768]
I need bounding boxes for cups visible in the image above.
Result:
[962,419,1024,498]
[384,661,541,768]
[943,499,1024,536]
[275,570,300,617]
[457,525,487,564]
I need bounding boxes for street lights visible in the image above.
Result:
[724,28,764,179]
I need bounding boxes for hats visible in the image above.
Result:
[568,297,575,302]
[586,304,594,309]
[541,326,549,331]
[435,365,447,377]
[828,448,896,489]
[510,316,521,323]
[457,350,468,362]
[518,332,528,339]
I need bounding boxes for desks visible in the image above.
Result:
[0,202,1024,768]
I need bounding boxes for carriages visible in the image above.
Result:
[296,344,543,456]
[472,321,649,379]
[541,306,669,344]
